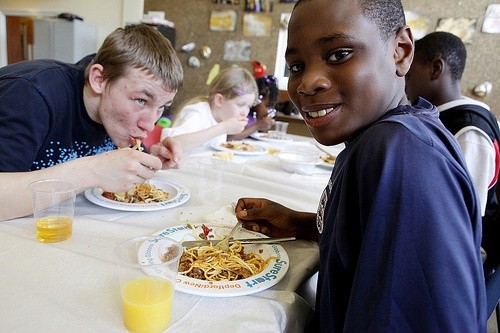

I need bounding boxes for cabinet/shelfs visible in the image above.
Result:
[33,18,98,64]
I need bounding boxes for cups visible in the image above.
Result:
[34,176,76,243]
[116,235,184,333]
[275,121,289,137]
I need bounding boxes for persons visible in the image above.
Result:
[227,75,279,142]
[234,0,488,333]
[0,24,183,222]
[405,31,500,283]
[160,66,259,155]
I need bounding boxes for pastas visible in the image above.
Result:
[161,219,278,282]
[102,139,170,204]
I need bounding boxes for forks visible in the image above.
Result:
[205,208,247,263]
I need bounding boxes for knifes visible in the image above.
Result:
[181,237,296,248]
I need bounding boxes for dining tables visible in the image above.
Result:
[0,127,347,333]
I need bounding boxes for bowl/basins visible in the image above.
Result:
[277,151,322,173]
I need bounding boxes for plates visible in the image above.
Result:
[84,179,190,212]
[250,132,292,142]
[139,222,290,297]
[317,152,337,168]
[210,142,270,154]
